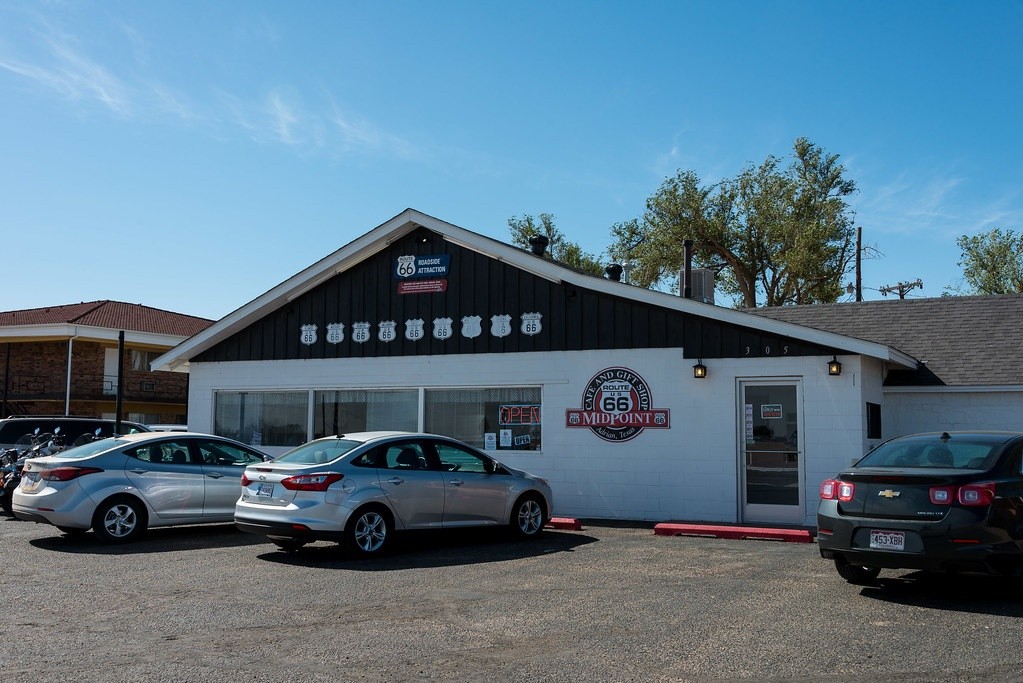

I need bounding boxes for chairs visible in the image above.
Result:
[927,447,956,465]
[312,450,329,463]
[363,454,381,465]
[171,449,187,464]
[148,445,165,462]
[394,447,424,469]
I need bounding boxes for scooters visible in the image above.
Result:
[0,426,107,518]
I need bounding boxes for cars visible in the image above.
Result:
[130,422,188,435]
[233,431,554,565]
[816,432,1023,610]
[11,432,278,547]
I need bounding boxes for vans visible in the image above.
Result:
[0,415,190,463]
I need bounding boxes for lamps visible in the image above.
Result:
[826,354,843,377]
[689,355,711,381]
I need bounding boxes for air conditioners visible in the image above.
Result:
[677,266,717,303]
